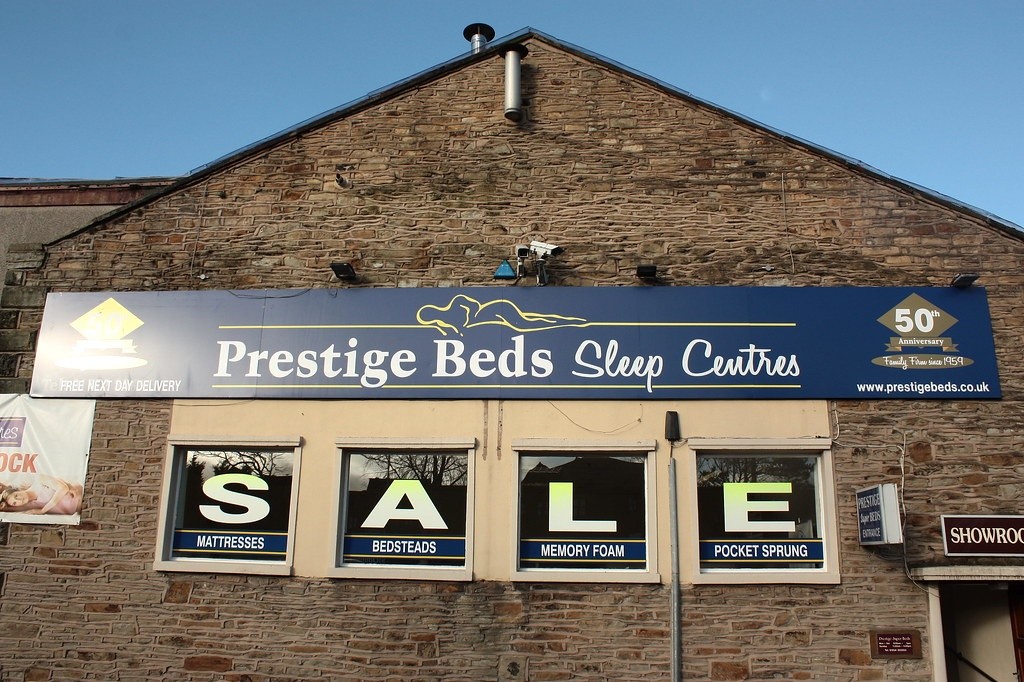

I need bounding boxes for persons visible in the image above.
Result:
[0,476,84,516]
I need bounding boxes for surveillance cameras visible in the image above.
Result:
[530,241,563,284]
[514,244,528,277]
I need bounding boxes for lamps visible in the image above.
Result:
[329,261,356,281]
[636,264,656,280]
[950,273,979,288]
